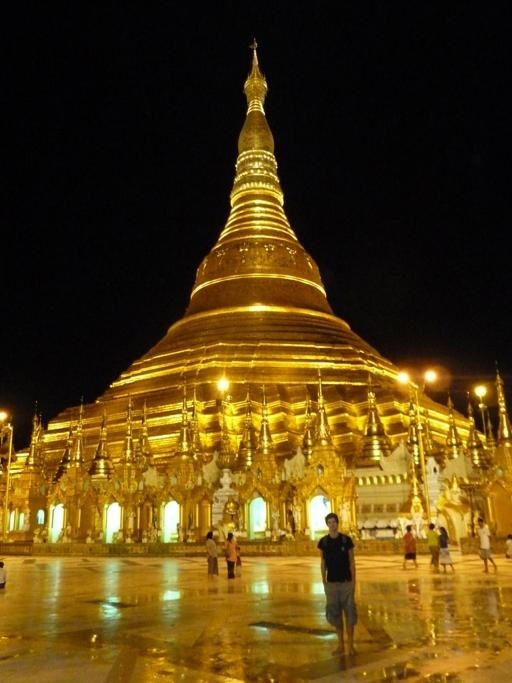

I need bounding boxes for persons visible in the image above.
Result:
[402,525,419,570]
[505,535,512,558]
[476,518,497,573]
[317,512,358,656]
[206,531,220,581]
[223,533,239,578]
[0,561,6,588]
[438,526,455,575]
[426,523,440,572]
